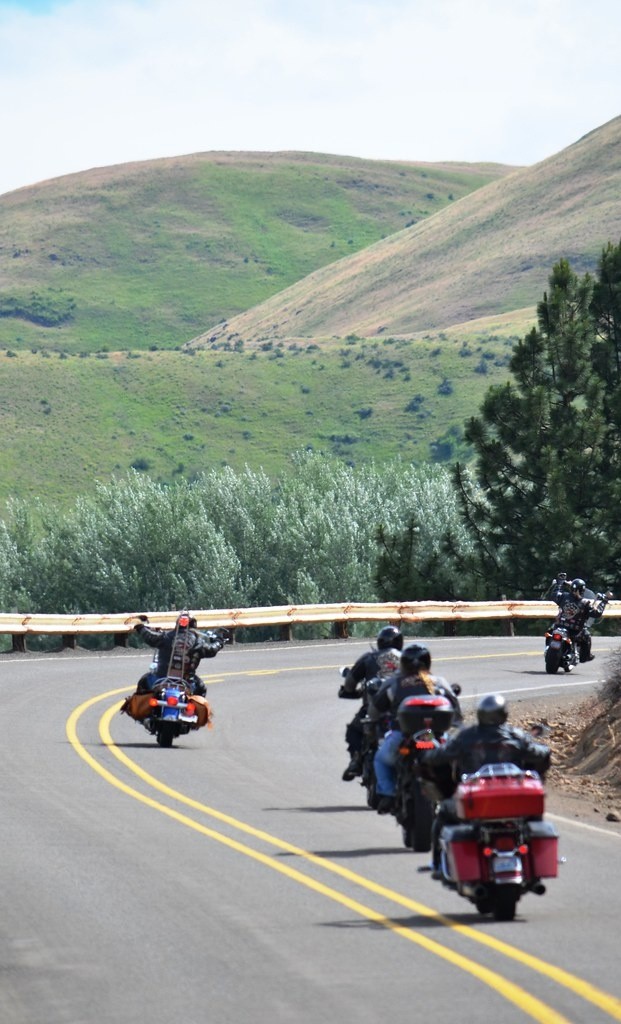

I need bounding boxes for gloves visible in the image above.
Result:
[134,624,144,633]
[214,638,225,649]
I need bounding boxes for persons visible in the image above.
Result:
[119,612,226,721]
[335,625,402,780]
[416,691,552,881]
[371,644,463,817]
[542,571,613,664]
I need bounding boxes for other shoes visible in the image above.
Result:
[343,752,361,781]
[580,654,594,663]
[378,795,395,815]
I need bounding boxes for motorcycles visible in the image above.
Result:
[543,573,613,675]
[124,614,230,748]
[338,665,394,809]
[413,721,559,922]
[359,678,462,852]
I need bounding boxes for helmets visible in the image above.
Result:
[377,625,404,651]
[401,643,431,673]
[176,614,197,629]
[570,579,586,592]
[476,694,507,725]
